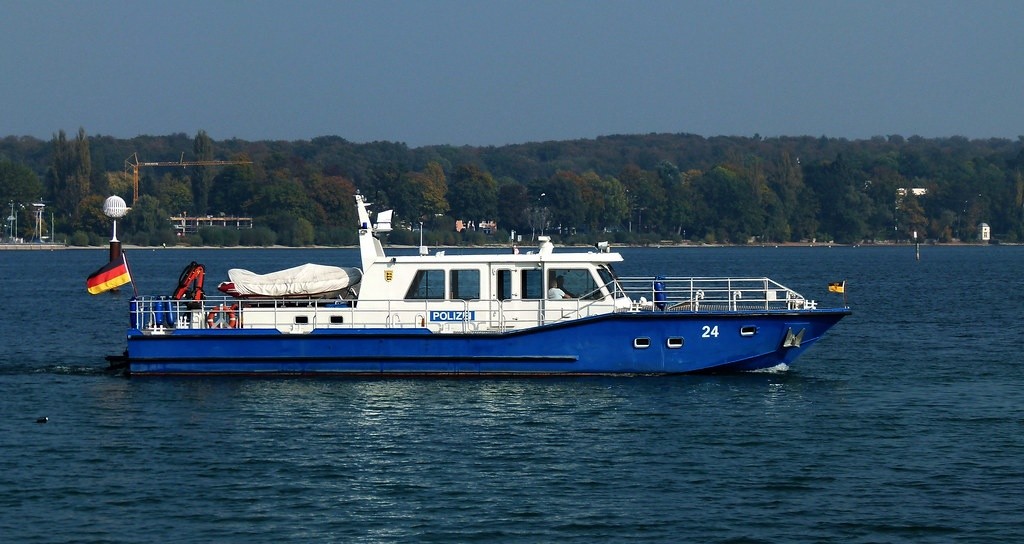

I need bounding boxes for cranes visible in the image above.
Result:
[123,150,254,210]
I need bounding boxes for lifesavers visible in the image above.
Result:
[206,305,236,329]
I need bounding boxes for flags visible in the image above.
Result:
[828,281,846,293]
[86,252,133,295]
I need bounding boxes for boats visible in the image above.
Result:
[216,265,362,300]
[102,188,855,385]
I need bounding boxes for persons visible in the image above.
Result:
[548,275,576,299]
